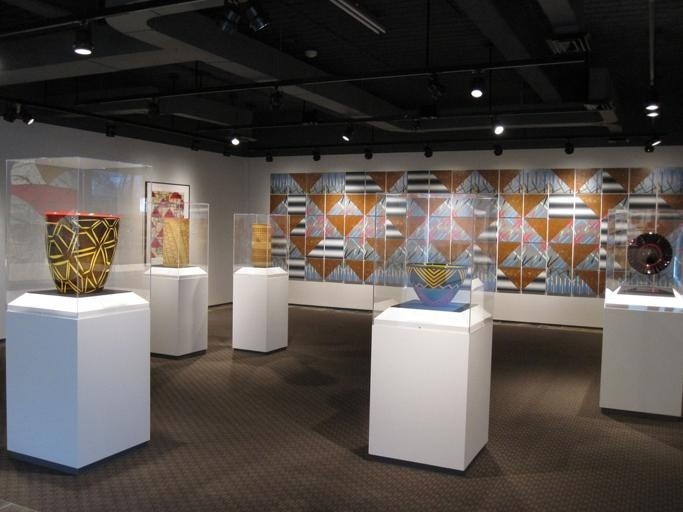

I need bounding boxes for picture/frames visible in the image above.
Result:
[140,177,191,268]
